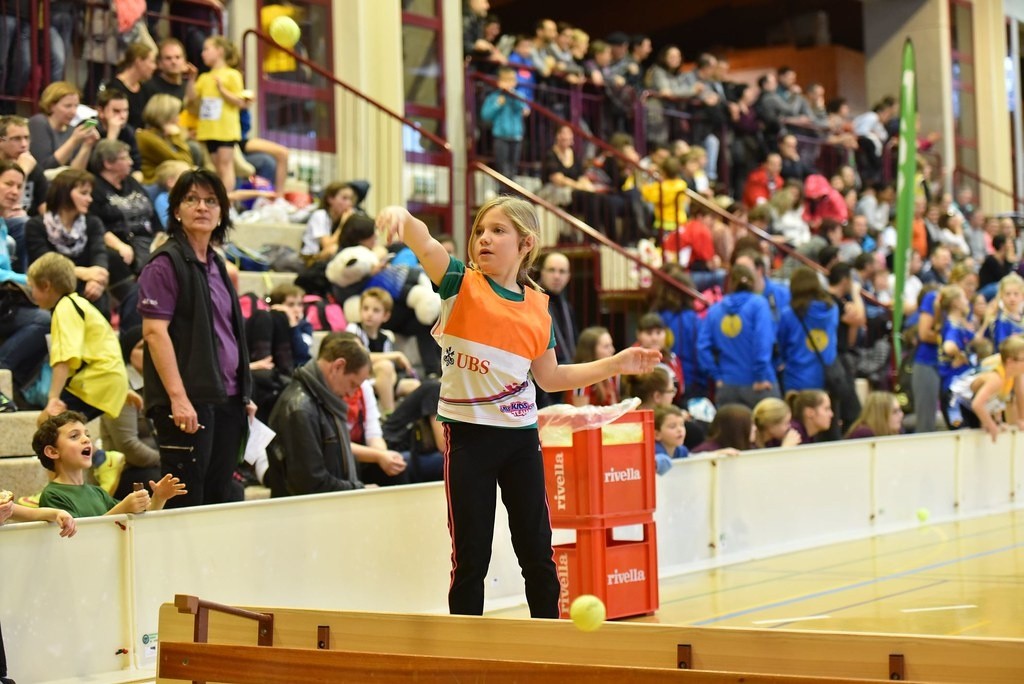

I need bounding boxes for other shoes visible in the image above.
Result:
[0,392,18,412]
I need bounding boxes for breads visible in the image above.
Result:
[0,490,14,505]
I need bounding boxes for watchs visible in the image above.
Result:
[777,265,839,392]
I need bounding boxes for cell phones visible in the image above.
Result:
[82,120,97,130]
[133,483,144,492]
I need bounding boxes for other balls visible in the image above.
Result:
[569,594,605,630]
[269,16,302,49]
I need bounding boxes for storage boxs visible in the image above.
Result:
[537,410,656,529]
[552,521,658,619]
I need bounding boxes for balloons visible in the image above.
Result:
[268,15,301,48]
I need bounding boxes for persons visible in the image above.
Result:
[538,125,620,244]
[375,197,664,620]
[480,68,530,197]
[30,411,188,519]
[135,169,259,510]
[535,252,579,404]
[347,286,422,416]
[181,36,252,192]
[742,152,784,211]
[653,405,689,460]
[0,0,1024,539]
[18,251,128,509]
[265,339,379,498]
[561,325,621,406]
[696,265,782,410]
[97,325,163,501]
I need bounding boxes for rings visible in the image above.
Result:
[180,423,185,430]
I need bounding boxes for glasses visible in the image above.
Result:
[665,387,677,394]
[183,195,219,208]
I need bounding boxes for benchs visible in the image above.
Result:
[474,172,660,302]
[1,224,423,503]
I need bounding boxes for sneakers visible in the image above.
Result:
[18,492,41,508]
[93,451,126,496]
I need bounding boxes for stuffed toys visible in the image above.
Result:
[324,246,442,326]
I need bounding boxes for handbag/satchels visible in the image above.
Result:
[239,293,347,336]
[261,244,298,272]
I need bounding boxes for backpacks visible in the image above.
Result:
[220,240,270,271]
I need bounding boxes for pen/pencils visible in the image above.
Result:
[168,414,205,430]
